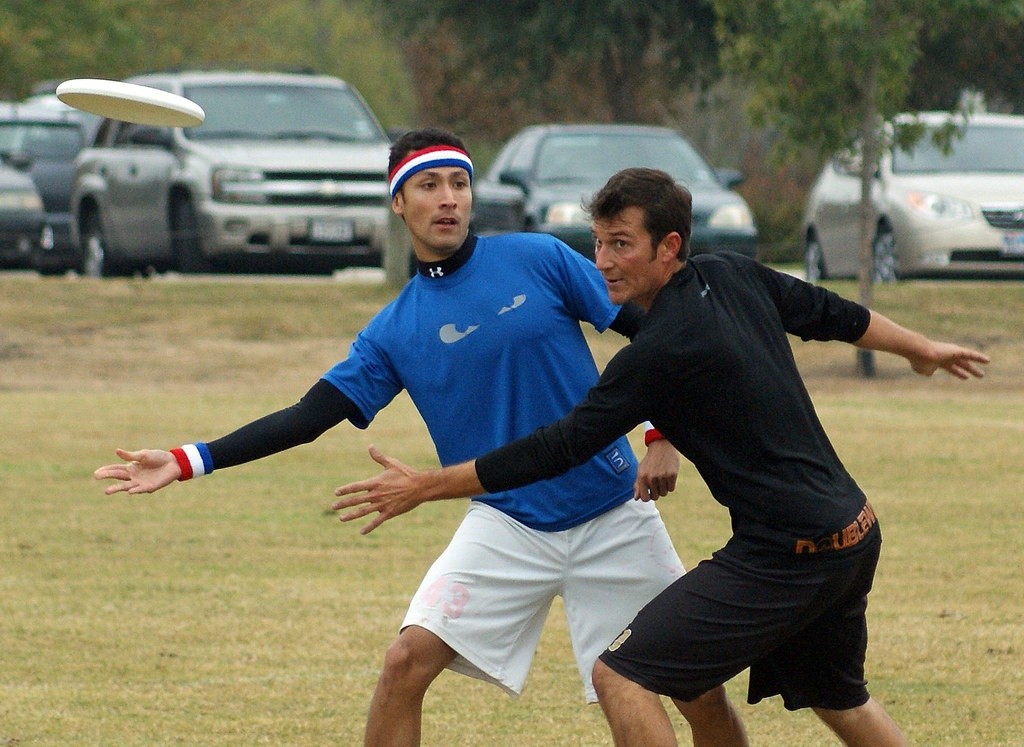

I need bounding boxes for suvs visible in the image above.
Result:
[69,68,402,277]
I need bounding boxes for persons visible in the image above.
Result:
[95,138,684,746]
[331,166,991,747]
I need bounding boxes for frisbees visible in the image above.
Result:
[55,79,206,128]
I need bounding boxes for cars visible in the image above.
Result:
[1,83,111,273]
[473,123,761,266]
[797,109,1024,285]
